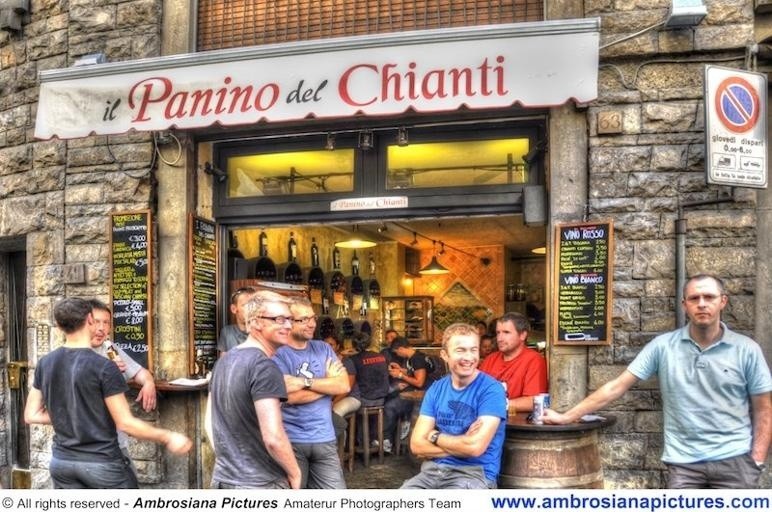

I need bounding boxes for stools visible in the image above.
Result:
[338,411,355,474]
[358,406,385,467]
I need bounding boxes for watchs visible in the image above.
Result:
[429,432,441,445]
[303,378,312,390]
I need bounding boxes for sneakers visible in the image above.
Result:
[400,421,411,440]
[372,438,392,453]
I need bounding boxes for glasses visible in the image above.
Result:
[256,315,295,324]
[232,288,247,301]
[295,314,318,322]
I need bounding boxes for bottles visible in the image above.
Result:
[405,326,423,333]
[321,289,328,314]
[342,294,350,317]
[352,249,360,275]
[332,242,340,270]
[540,394,550,409]
[311,237,319,265]
[532,396,543,425]
[369,253,375,274]
[507,283,515,300]
[406,332,424,338]
[363,298,367,315]
[194,350,206,380]
[515,284,524,300]
[406,316,424,323]
[288,232,297,262]
[500,380,509,418]
[259,229,267,256]
[229,230,238,247]
[407,305,418,313]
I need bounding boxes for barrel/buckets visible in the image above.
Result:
[498,429,604,489]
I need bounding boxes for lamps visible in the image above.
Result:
[419,257,449,274]
[667,1,708,27]
[335,232,376,248]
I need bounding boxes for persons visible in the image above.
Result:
[62,298,155,477]
[348,333,389,407]
[204,291,301,489]
[479,312,547,412]
[399,322,507,489]
[218,287,257,357]
[372,336,435,455]
[380,329,411,440]
[322,333,361,448]
[480,335,494,361]
[537,274,772,489]
[476,321,487,337]
[23,297,192,489]
[272,292,351,489]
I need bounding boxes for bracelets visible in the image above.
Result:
[399,372,403,378]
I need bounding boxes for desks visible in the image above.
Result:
[505,410,615,431]
[126,376,207,391]
[398,391,423,400]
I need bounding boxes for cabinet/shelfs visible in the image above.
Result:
[382,296,434,344]
